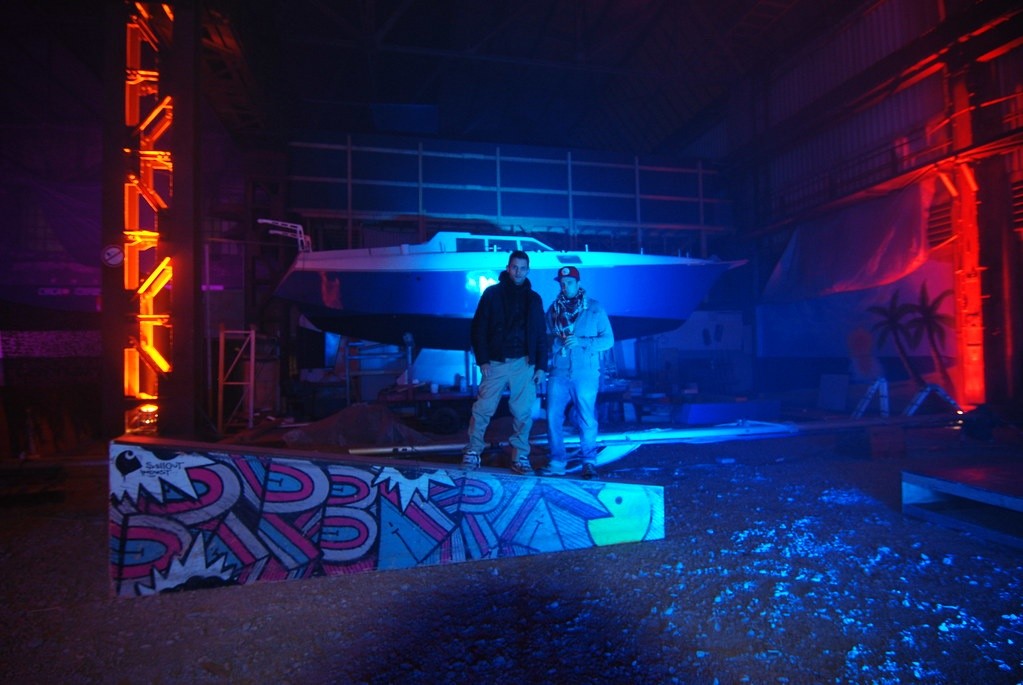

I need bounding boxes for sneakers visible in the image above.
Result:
[461,450,481,471]
[581,462,600,481]
[537,459,567,476]
[511,455,534,475]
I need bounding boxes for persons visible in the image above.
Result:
[545,266,614,480]
[459,250,548,475]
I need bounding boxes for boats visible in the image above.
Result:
[257,217,747,352]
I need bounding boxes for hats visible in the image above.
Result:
[553,266,580,282]
